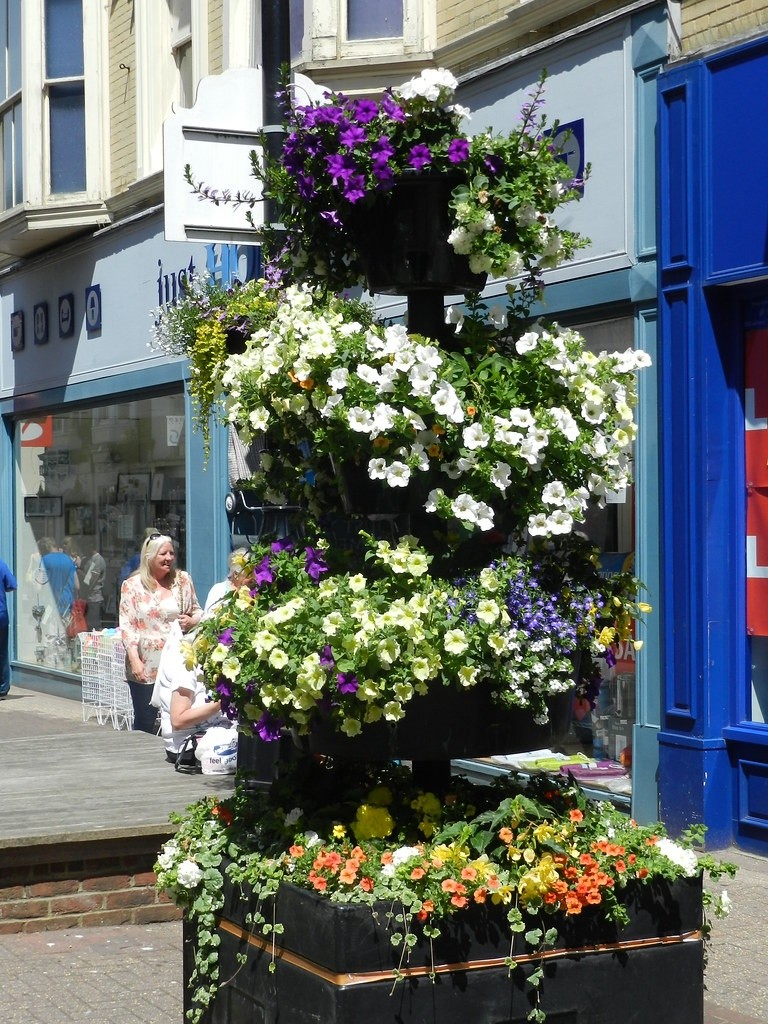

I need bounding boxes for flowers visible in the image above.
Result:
[151,67,742,1024]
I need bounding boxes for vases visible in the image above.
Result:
[181,842,704,1024]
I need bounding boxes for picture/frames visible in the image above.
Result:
[24,473,164,534]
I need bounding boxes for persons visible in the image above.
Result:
[0,560,18,699]
[200,546,256,624]
[28,531,142,669]
[160,623,235,764]
[118,525,203,734]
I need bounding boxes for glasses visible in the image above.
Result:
[145,533,174,546]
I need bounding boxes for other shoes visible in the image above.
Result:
[0,685,11,699]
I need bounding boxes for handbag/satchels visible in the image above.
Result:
[66,595,87,638]
